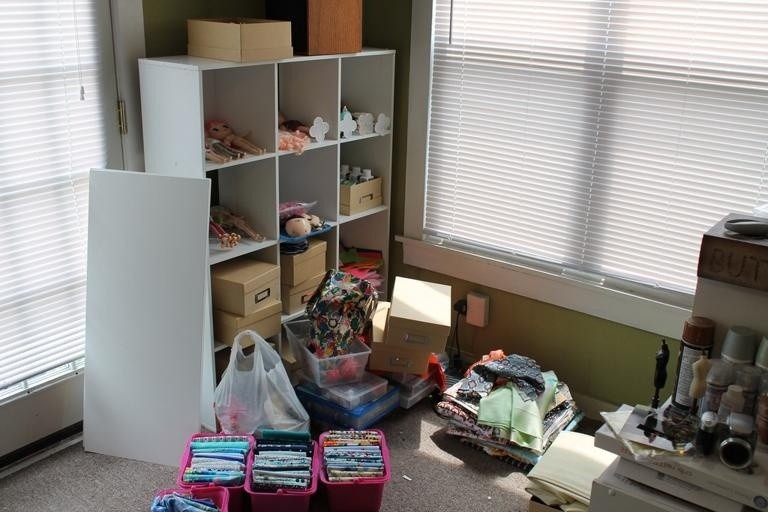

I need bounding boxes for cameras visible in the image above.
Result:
[696,411,759,470]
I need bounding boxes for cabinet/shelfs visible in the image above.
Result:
[137,45,397,432]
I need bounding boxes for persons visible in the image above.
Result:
[203,112,327,250]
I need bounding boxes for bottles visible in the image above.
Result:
[673,317,767,469]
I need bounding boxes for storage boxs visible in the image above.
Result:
[284,317,374,392]
[279,239,328,288]
[281,270,328,315]
[367,298,429,379]
[340,175,384,216]
[186,1,363,63]
[149,485,231,511]
[210,258,282,317]
[383,274,452,356]
[319,429,394,510]
[211,297,283,351]
[288,354,453,429]
[175,432,254,491]
[243,434,321,511]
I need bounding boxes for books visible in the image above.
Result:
[588,403,768,511]
